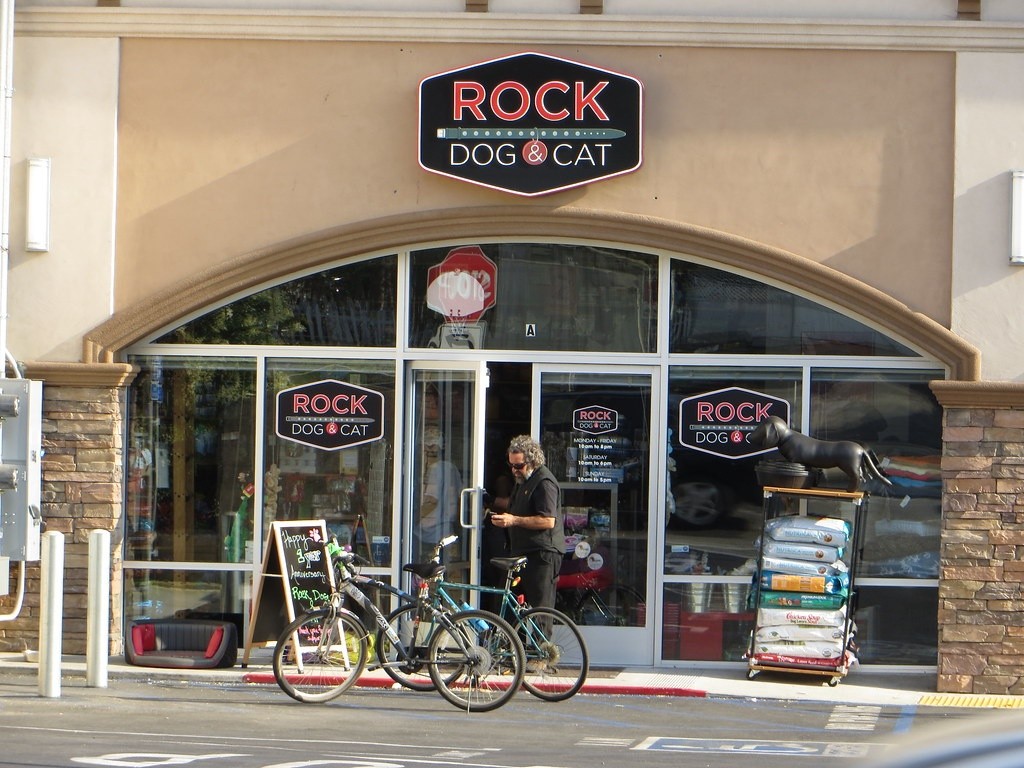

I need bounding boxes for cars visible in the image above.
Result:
[668,438,766,532]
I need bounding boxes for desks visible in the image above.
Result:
[681,611,754,661]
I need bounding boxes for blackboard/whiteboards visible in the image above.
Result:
[247,519,346,654]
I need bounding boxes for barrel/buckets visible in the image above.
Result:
[682,583,714,612]
[721,583,750,614]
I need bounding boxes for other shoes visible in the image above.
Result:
[524,658,546,672]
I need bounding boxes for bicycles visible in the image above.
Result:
[552,568,647,629]
[374,534,590,702]
[273,527,526,716]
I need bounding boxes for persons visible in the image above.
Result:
[491,435,564,677]
[419,429,462,568]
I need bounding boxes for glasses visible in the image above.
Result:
[507,460,531,469]
